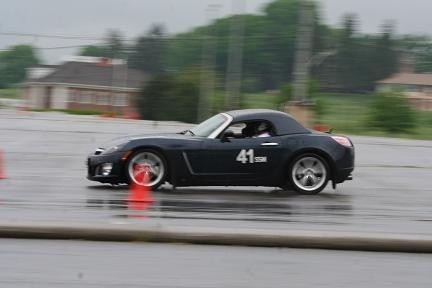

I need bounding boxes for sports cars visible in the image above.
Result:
[87,109,354,196]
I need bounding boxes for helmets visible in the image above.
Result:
[254,120,269,132]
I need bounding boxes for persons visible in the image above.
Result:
[224,120,273,138]
